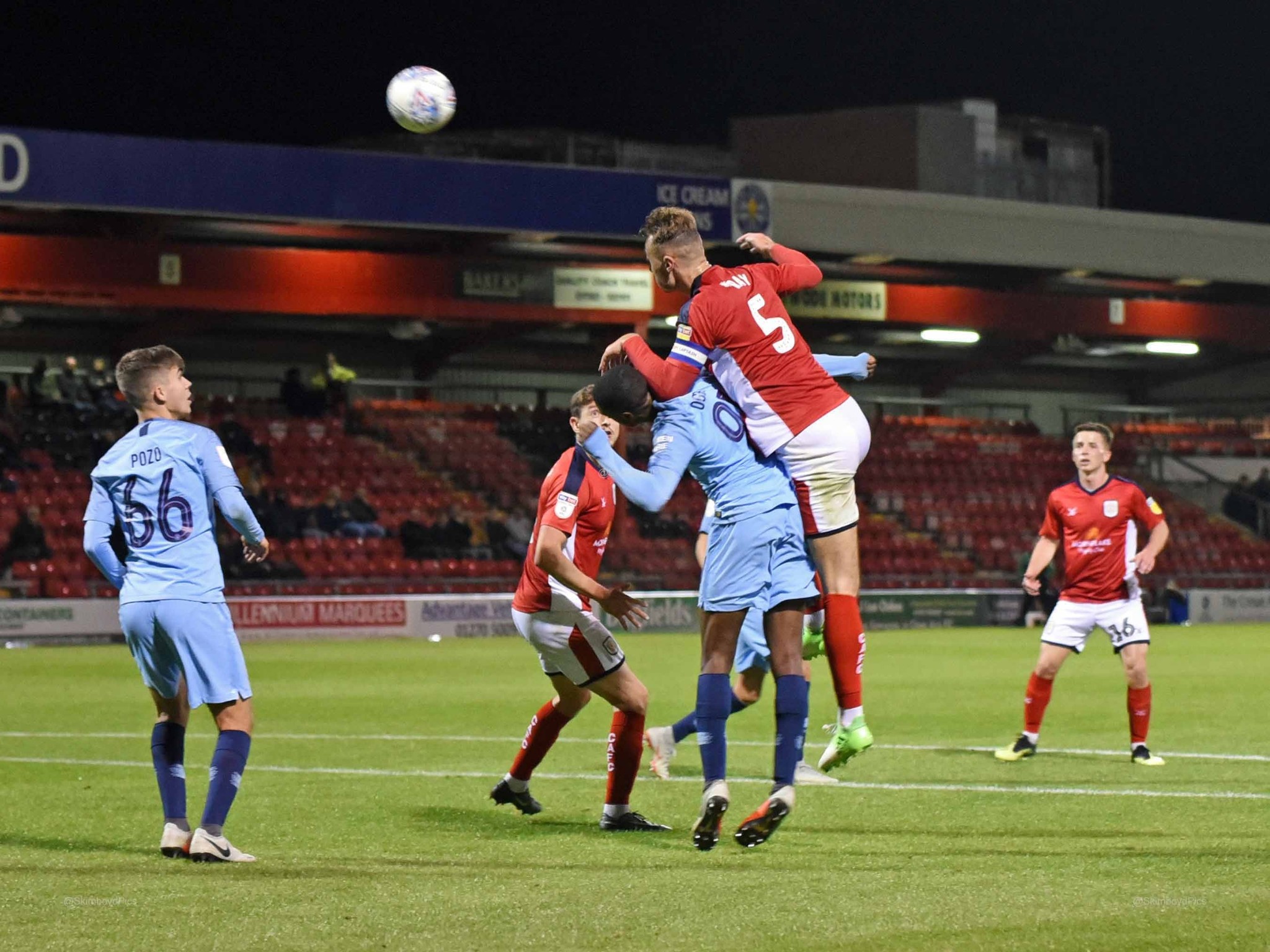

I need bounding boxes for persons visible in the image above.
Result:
[84,345,269,863]
[1223,469,1270,534]
[995,422,1169,768]
[1011,538,1054,627]
[0,353,696,584]
[578,206,876,851]
[490,383,672,833]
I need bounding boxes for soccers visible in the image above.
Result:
[386,65,458,135]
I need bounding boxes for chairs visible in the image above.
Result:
[0,385,1270,603]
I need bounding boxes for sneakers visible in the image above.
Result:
[189,827,256,863]
[690,780,730,851]
[599,810,673,831]
[802,624,826,660]
[793,759,838,787]
[490,780,542,815]
[734,786,795,848]
[643,725,676,780]
[159,821,188,855]
[817,707,874,773]
[994,733,1038,762]
[1131,745,1165,766]
